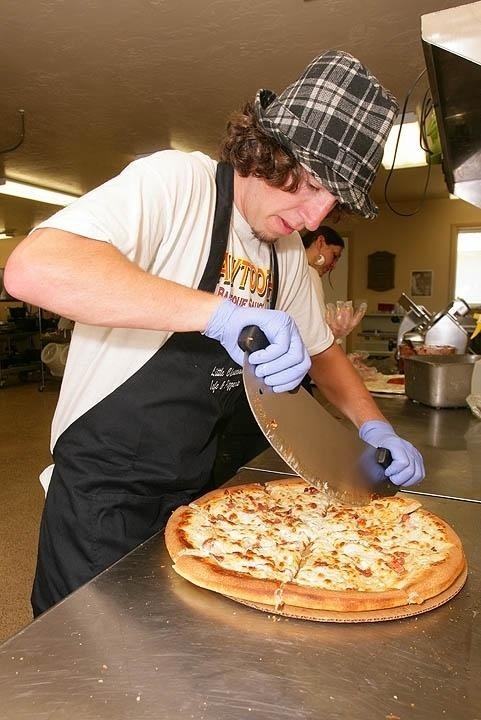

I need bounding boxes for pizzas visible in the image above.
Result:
[164,477,465,612]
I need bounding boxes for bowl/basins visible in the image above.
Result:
[7,306,27,317]
[377,302,396,313]
[397,344,459,374]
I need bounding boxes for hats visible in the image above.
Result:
[253,45,404,222]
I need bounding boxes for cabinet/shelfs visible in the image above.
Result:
[38,307,72,393]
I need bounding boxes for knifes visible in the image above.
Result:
[238,324,404,507]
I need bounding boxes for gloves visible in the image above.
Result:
[198,297,313,398]
[356,416,425,489]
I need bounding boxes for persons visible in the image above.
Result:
[3,48,427,621]
[302,225,369,396]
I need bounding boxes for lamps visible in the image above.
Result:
[381,111,430,170]
[0,177,80,208]
